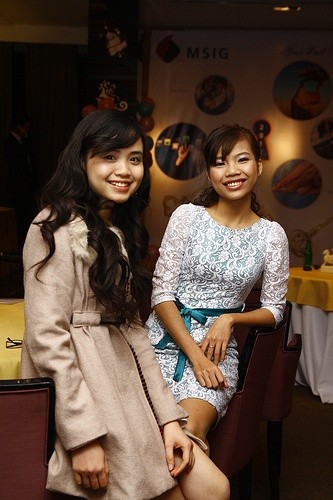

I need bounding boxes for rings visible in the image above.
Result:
[209,346,214,348]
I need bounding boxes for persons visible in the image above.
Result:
[20,109,229,500]
[144,123,289,458]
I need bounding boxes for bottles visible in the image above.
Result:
[303,239,313,271]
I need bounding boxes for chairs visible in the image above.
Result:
[208,285,302,500]
[0,377,64,500]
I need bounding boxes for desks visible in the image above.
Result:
[285,266,333,405]
[0,295,25,380]
